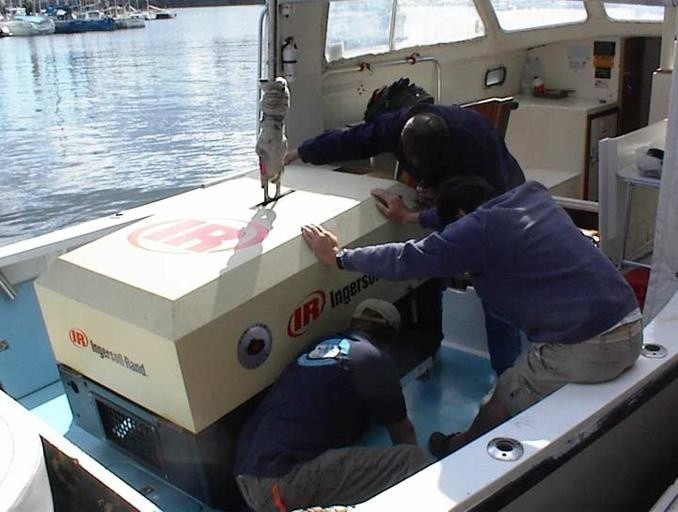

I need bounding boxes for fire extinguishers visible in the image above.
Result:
[281,37,298,83]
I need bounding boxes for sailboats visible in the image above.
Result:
[0,0,176,37]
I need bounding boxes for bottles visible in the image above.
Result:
[520,58,545,98]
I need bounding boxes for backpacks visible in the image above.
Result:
[362,77,436,123]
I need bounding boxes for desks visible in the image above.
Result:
[617,163,661,271]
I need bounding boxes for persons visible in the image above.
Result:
[300,171,646,462]
[230,296,432,512]
[281,101,530,380]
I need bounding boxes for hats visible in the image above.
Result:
[401,112,450,187]
[352,297,410,344]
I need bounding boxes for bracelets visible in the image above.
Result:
[335,247,344,272]
